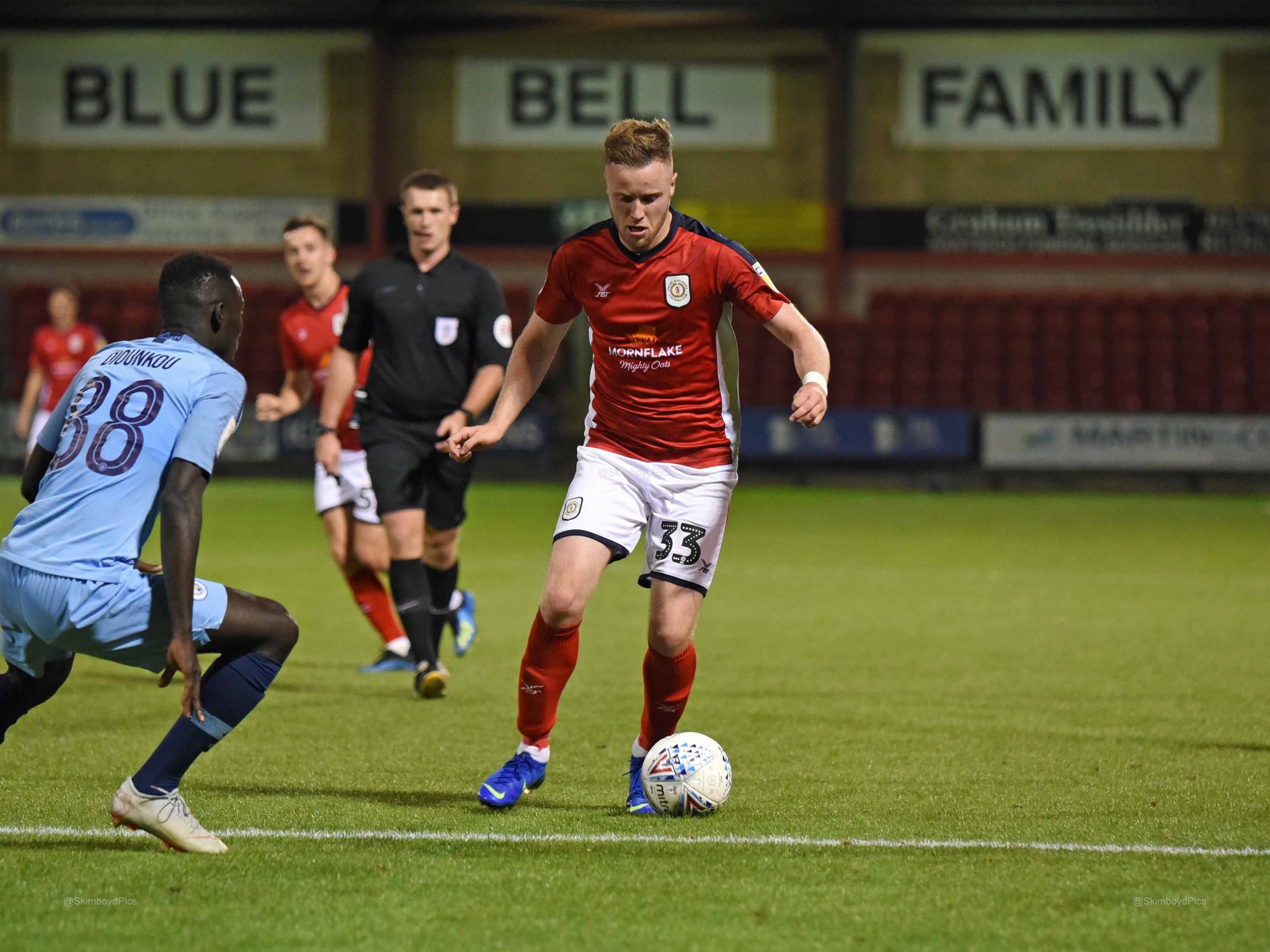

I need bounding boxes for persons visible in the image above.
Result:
[447,120,830,814]
[255,216,479,674]
[16,284,108,463]
[0,252,299,853]
[316,171,513,699]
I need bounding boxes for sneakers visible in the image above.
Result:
[626,754,656,815]
[110,777,227,853]
[450,590,477,654]
[362,645,414,674]
[478,752,547,811]
[415,662,449,699]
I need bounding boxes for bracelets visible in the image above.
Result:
[802,371,828,396]
[460,408,472,424]
[316,425,334,436]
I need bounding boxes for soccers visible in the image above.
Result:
[640,731,733,817]
